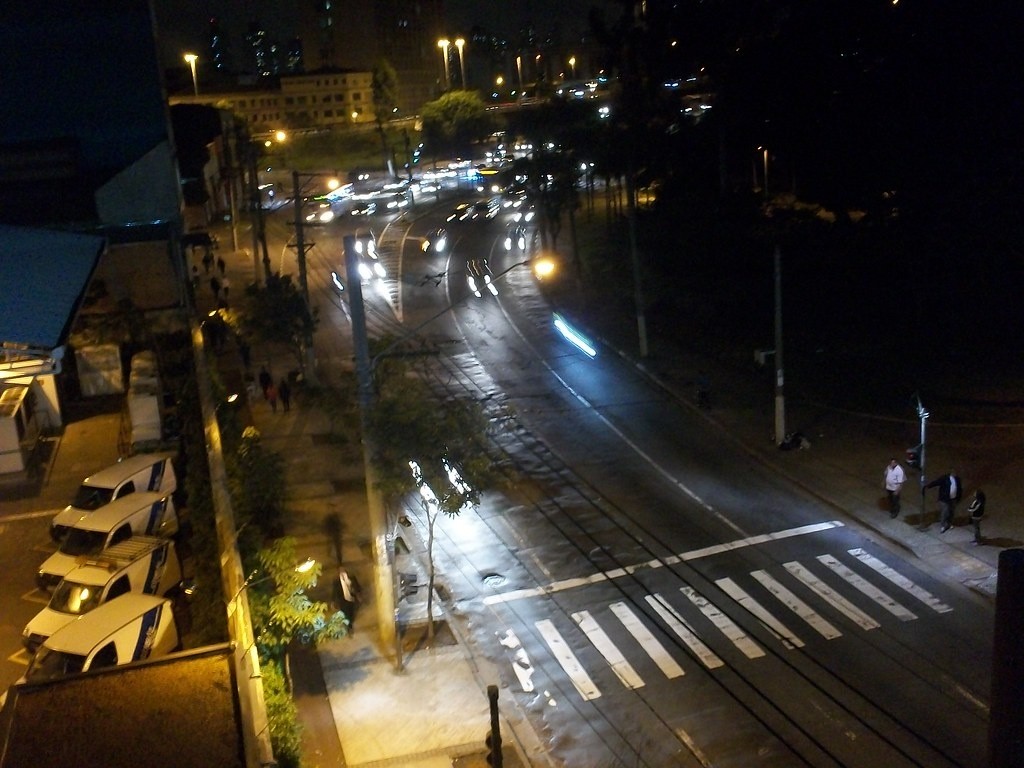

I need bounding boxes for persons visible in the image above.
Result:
[266,381,278,414]
[259,365,273,400]
[190,245,230,308]
[967,489,986,545]
[330,563,363,635]
[319,502,348,565]
[881,458,907,518]
[278,376,291,411]
[924,467,963,533]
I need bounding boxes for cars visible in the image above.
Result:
[255,95,614,323]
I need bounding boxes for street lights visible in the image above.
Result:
[438,36,451,92]
[455,34,467,91]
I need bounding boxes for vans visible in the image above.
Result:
[35,491,181,594]
[49,452,187,540]
[21,535,184,653]
[0,589,183,714]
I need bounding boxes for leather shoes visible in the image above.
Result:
[889,510,899,518]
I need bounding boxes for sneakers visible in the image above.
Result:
[940,524,954,533]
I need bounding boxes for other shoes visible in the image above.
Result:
[969,537,984,546]
